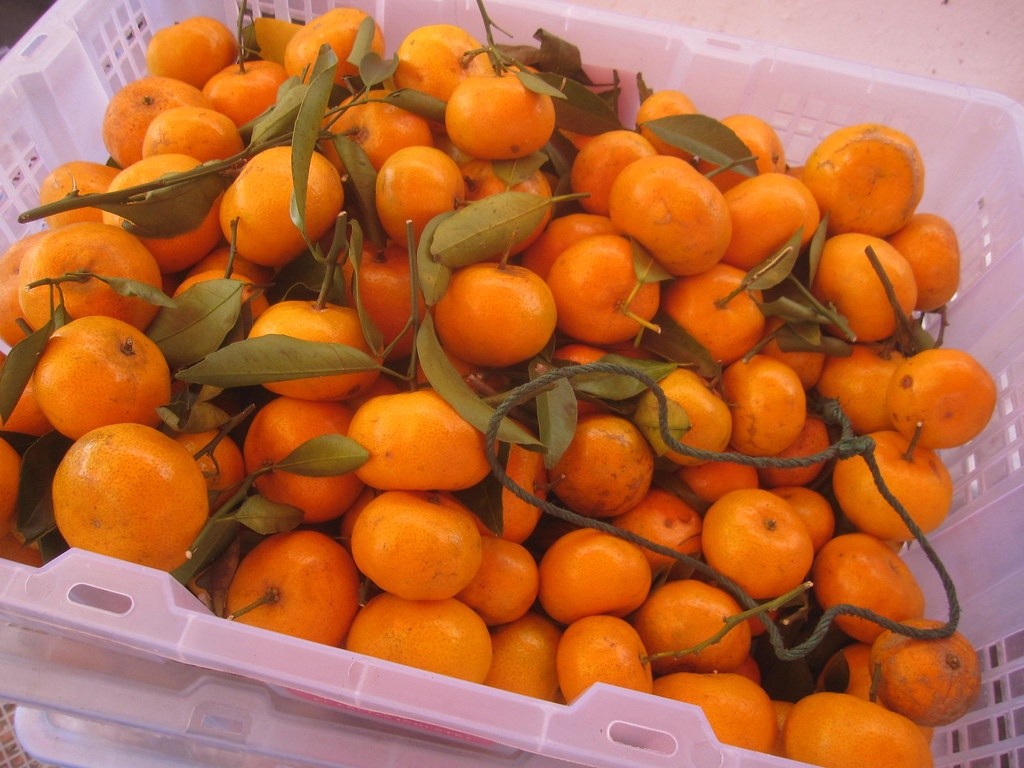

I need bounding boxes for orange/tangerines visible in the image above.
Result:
[0,8,998,768]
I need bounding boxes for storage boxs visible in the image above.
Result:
[1,0,1024,768]
